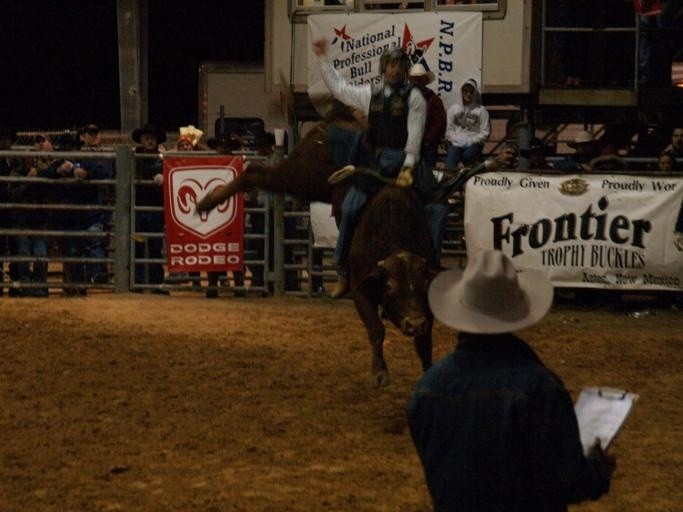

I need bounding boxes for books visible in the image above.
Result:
[574,385,639,461]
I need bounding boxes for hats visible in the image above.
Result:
[568,130,601,151]
[409,63,436,84]
[426,248,556,335]
[519,137,553,160]
[35,124,274,150]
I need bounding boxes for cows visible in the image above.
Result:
[189,90,442,389]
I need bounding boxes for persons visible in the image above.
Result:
[308,37,451,296]
[441,80,491,159]
[403,251,621,510]
[0,120,681,306]
[411,62,448,165]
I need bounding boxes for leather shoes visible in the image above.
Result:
[329,279,350,299]
